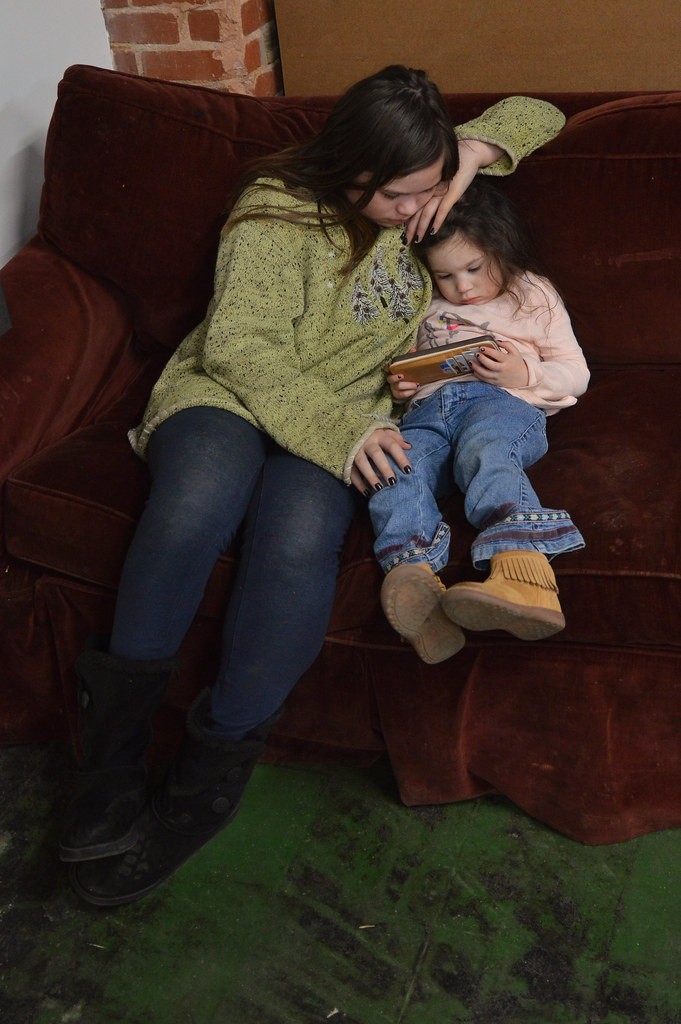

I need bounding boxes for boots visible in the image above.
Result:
[440,551,567,644]
[379,563,467,665]
[71,681,289,908]
[56,646,173,863]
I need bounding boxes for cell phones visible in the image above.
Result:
[389,335,500,385]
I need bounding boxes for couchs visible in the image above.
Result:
[0,64,681,847]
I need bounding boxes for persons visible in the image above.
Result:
[371,175,587,664]
[26,64,571,907]
